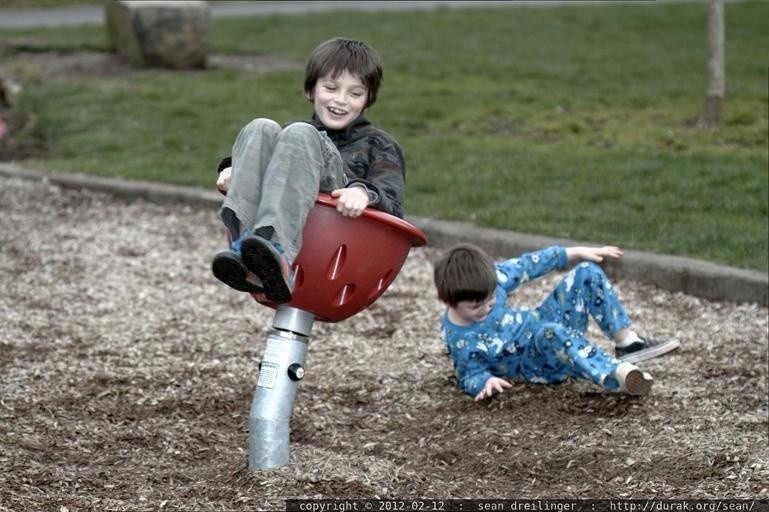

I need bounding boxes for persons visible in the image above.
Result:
[434,242,681,405]
[211,36,406,305]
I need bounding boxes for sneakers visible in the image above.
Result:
[614,334,681,364]
[617,363,652,396]
[211,234,291,307]
